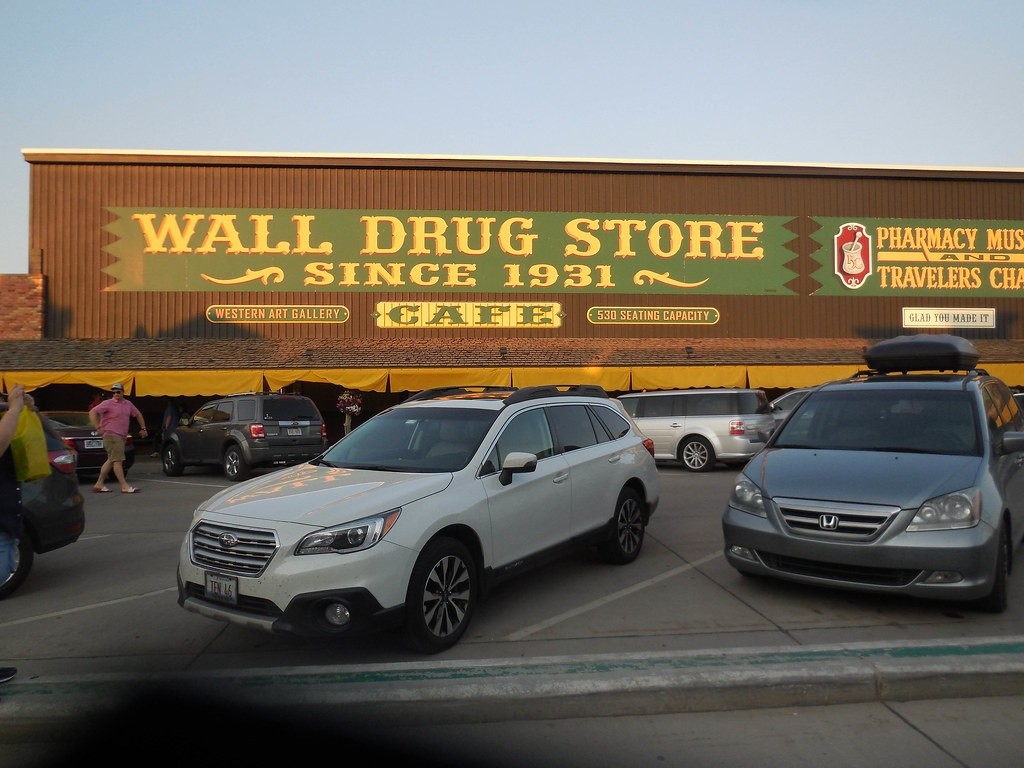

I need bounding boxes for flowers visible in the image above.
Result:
[336,389,365,416]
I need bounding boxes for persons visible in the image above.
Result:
[89,383,148,493]
[0,384,37,588]
[150,396,190,457]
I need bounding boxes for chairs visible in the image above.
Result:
[425,420,470,457]
[909,406,977,454]
[827,403,887,448]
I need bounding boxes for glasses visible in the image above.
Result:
[112,391,121,394]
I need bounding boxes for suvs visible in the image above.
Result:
[173,382,662,650]
[159,391,329,481]
[719,332,1024,613]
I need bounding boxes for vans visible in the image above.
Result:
[614,388,775,471]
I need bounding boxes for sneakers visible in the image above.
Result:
[0,667,17,683]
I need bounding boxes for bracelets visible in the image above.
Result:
[141,427,146,430]
[96,426,101,430]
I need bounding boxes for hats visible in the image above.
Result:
[111,383,124,391]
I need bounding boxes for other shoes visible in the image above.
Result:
[150,452,159,457]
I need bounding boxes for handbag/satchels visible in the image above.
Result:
[0,404,52,483]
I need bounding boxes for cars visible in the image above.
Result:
[36,408,135,480]
[0,405,86,598]
[768,384,818,437]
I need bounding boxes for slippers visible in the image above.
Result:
[121,486,140,493]
[92,486,113,493]
